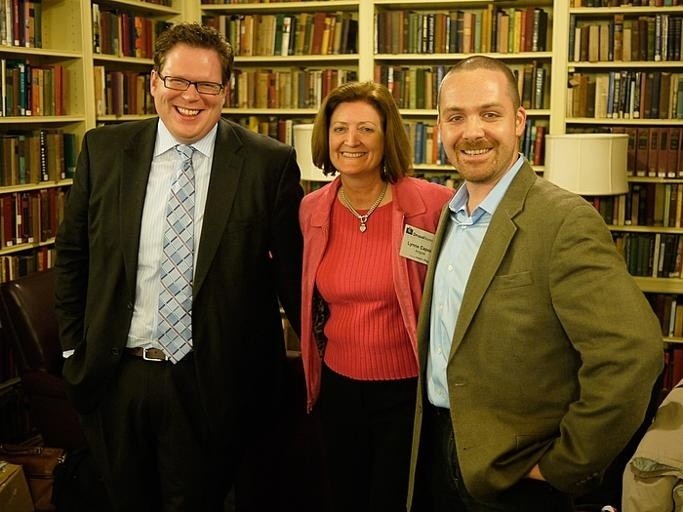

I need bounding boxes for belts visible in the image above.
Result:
[128,344,169,365]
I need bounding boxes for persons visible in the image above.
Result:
[47,21,307,511]
[295,79,458,511]
[400,52,666,511]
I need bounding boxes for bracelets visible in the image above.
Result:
[57,343,78,362]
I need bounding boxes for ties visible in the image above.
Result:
[155,145,199,366]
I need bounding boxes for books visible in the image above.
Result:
[646,286,683,394]
[374,2,554,192]
[566,1,682,279]
[90,1,174,128]
[1,1,80,282]
[201,0,358,197]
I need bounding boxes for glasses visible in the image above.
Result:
[155,73,226,97]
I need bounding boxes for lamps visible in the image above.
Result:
[544,132,631,197]
[292,123,340,182]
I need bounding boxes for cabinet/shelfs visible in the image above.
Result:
[374,0,553,193]
[92,0,186,127]
[195,0,361,357]
[560,0,683,343]
[0,0,97,282]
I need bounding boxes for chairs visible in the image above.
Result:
[0,267,78,449]
